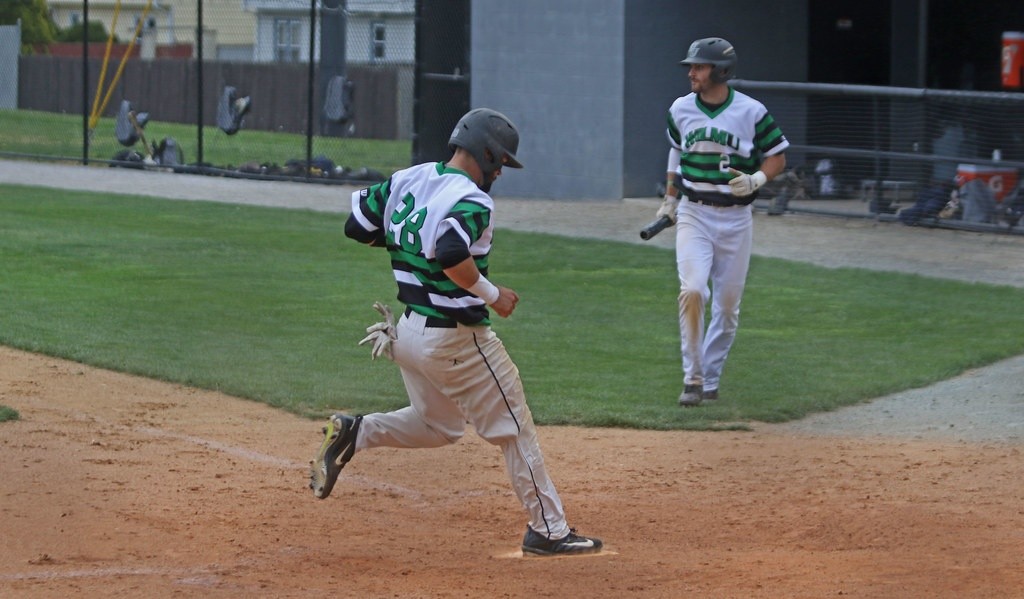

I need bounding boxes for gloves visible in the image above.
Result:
[728,168,768,197]
[656,195,678,227]
[358,301,398,361]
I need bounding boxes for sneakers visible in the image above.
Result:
[698,389,720,405]
[522,524,603,557]
[679,384,704,405]
[309,413,363,499]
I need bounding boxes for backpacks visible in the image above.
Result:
[216,84,252,135]
[152,137,183,171]
[116,97,149,147]
[958,179,999,232]
[321,75,357,124]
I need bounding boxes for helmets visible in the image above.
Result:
[448,108,524,193]
[680,38,737,84]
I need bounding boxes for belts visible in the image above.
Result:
[405,305,459,328]
[684,192,756,207]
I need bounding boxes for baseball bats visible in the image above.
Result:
[639,209,677,241]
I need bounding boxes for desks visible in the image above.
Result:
[862,179,918,205]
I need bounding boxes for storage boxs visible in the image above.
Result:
[957,164,1017,202]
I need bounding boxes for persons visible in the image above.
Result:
[309,107,603,557]
[655,37,791,408]
